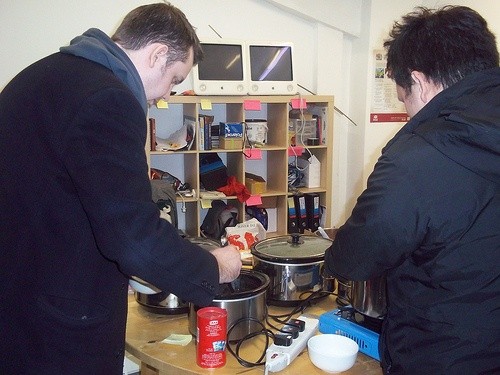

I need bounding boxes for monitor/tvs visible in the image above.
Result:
[192,40,298,95]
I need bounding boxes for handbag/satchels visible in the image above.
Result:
[202,200,239,242]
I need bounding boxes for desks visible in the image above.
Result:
[125,278,383,375]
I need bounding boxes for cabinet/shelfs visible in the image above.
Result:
[144,94,334,238]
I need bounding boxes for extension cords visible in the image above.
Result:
[265,316,319,372]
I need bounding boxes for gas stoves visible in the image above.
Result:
[318,305,381,363]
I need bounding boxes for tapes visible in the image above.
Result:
[306,137,320,146]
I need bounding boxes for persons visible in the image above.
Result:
[0,0,243,375]
[323,3,500,375]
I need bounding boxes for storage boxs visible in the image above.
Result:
[297,157,320,189]
[220,122,242,138]
[290,118,317,146]
[219,138,243,150]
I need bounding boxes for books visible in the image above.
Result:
[199,113,221,151]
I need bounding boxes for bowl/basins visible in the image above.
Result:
[308,333,359,373]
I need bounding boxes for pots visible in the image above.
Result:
[134,236,222,315]
[250,234,336,307]
[188,270,270,344]
[336,277,353,307]
[351,276,386,320]
